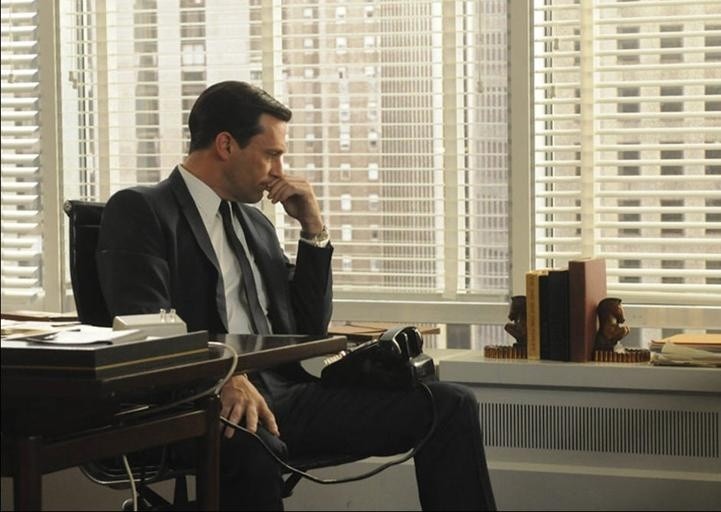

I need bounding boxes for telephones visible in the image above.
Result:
[321,326,424,392]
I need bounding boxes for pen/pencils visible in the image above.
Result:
[52,322,82,326]
[70,329,81,331]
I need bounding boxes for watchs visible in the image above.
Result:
[300,224,330,241]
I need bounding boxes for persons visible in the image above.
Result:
[95,81,498,511]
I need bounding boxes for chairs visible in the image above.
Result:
[62,199,376,510]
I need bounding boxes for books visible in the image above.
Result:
[648,333,721,368]
[1,322,148,348]
[526,257,607,363]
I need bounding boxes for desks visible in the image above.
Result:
[0,331,349,511]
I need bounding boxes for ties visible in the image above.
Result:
[218,200,270,335]
